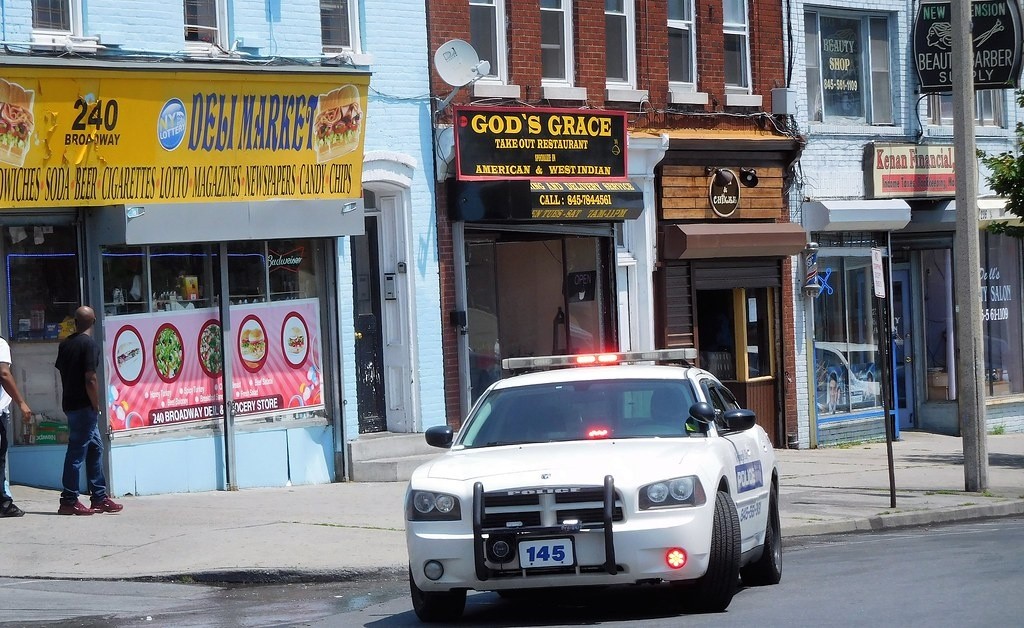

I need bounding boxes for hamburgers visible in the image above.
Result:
[286,327,305,355]
[240,329,265,358]
[116,341,140,367]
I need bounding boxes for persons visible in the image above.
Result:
[0,336,32,518]
[54,305,123,516]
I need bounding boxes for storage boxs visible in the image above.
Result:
[927,370,948,401]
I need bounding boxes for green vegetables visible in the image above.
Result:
[202,326,223,373]
[155,327,182,377]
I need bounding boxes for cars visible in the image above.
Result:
[405,347,783,624]
[812,344,946,422]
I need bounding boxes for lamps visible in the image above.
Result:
[704,166,733,187]
[739,166,758,188]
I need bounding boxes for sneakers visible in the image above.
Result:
[90,494,123,512]
[58,498,95,515]
[0,501,25,518]
